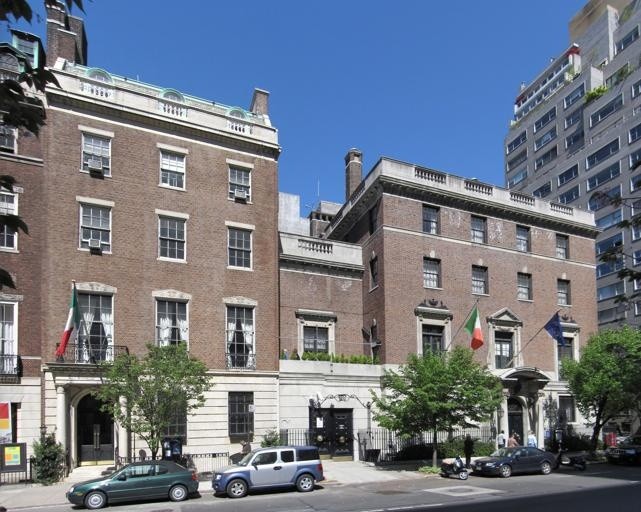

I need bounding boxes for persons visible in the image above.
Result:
[497,429,507,449]
[508,432,521,452]
[465,434,474,469]
[239,439,252,455]
[529,430,540,448]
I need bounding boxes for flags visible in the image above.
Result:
[545,313,568,348]
[55,284,84,357]
[463,305,485,351]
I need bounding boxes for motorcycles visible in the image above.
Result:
[441,451,468,480]
[556,444,587,473]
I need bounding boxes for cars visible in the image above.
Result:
[470,446,559,478]
[609,436,640,466]
[213,446,325,498]
[66,461,199,510]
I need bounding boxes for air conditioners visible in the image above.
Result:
[87,158,104,176]
[234,189,247,200]
[88,238,101,250]
